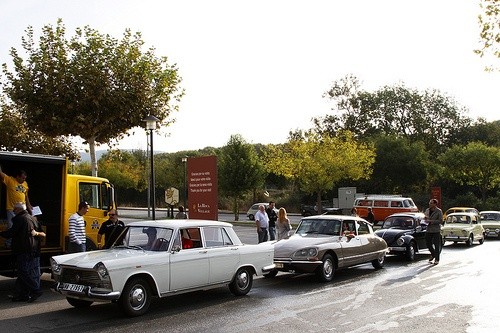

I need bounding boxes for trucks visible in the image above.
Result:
[0,151,117,279]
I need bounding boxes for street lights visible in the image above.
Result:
[181,154,188,215]
[142,115,160,221]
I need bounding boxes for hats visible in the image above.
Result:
[143,228,157,233]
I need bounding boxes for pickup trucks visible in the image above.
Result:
[299,200,343,217]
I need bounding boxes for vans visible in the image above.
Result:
[353,197,419,225]
[443,207,479,222]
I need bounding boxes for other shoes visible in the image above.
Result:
[7,294,25,301]
[434,261,438,265]
[27,291,41,303]
[429,256,434,261]
[5,240,11,248]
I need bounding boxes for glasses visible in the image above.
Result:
[108,213,117,217]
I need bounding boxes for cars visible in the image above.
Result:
[440,212,485,247]
[258,215,389,282]
[247,203,279,221]
[373,212,443,261]
[50,219,275,316]
[479,211,500,239]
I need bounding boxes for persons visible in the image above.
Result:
[97,210,126,250]
[267,201,278,241]
[254,204,269,244]
[350,208,359,217]
[142,228,161,250]
[275,207,292,240]
[366,208,375,223]
[425,199,443,265]
[49,202,90,295]
[180,229,193,250]
[483,215,488,220]
[313,204,319,214]
[175,205,187,218]
[0,166,47,303]
